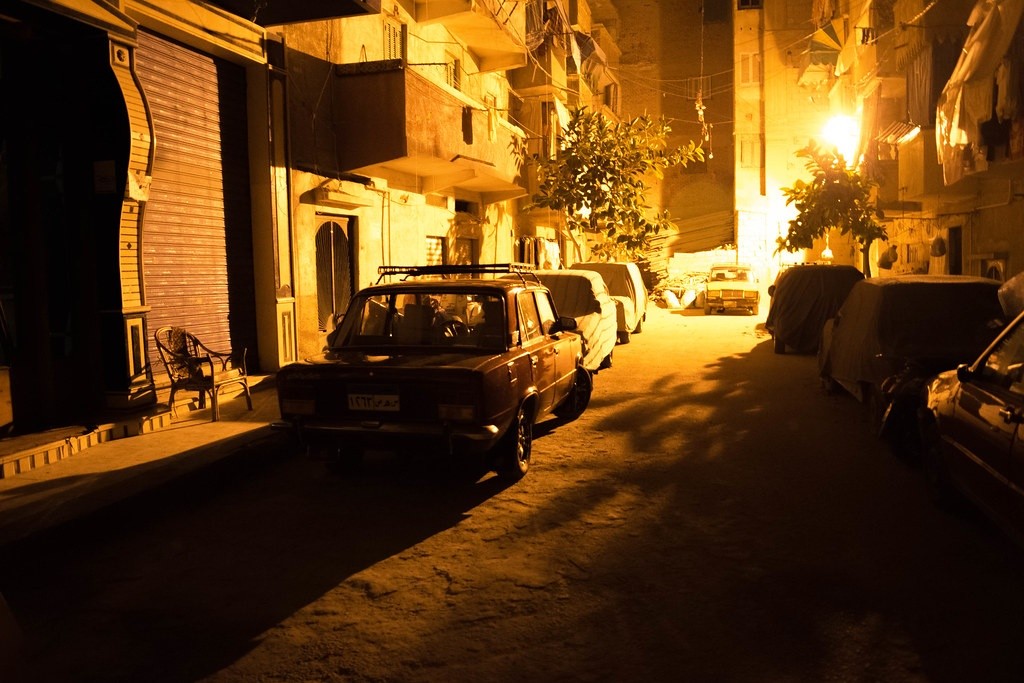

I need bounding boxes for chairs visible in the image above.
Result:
[155,326,254,422]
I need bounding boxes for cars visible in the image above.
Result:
[704,264,760,316]
[763,265,1024,546]
[270,261,647,480]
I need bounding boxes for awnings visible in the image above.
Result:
[797,13,851,86]
[571,31,606,93]
[894,0,968,73]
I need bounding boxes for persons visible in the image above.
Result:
[425,298,447,323]
[467,296,485,323]
